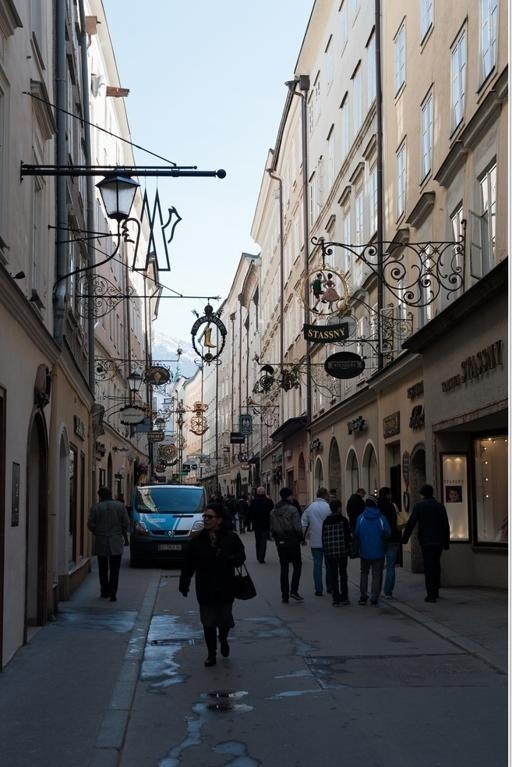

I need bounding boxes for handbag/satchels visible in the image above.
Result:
[234,553,256,600]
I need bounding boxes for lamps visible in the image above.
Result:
[103,371,143,412]
[20,160,227,298]
[154,417,166,431]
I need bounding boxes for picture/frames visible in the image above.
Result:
[440,451,472,544]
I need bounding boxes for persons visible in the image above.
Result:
[309,272,327,313]
[397,485,451,605]
[321,272,339,312]
[116,487,400,607]
[178,499,246,669]
[446,490,462,503]
[88,486,129,602]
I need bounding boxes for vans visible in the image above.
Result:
[130,482,211,565]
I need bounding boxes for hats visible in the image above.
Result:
[256,487,265,495]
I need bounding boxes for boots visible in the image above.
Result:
[205,649,216,666]
[218,635,229,657]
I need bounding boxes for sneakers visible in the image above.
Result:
[339,598,350,604]
[370,599,377,607]
[359,598,366,604]
[290,590,303,600]
[332,601,339,606]
[385,591,392,599]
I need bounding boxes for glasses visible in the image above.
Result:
[202,513,218,519]
